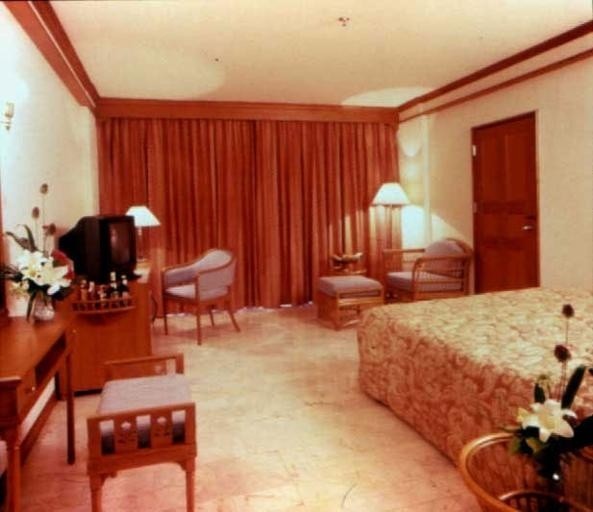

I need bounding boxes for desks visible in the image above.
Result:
[1,317,75,509]
[459,433,593,511]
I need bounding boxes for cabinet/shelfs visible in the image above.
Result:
[55,259,158,401]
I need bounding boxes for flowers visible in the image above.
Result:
[495,304,593,497]
[3,182,74,324]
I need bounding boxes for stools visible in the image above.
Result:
[86,352,197,512]
[317,275,384,331]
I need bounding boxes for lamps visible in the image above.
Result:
[125,206,162,262]
[369,181,411,250]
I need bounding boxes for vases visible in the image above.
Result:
[531,458,566,501]
[32,294,55,321]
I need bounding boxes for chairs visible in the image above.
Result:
[160,248,241,346]
[381,238,473,302]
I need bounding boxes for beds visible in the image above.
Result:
[357,287,593,506]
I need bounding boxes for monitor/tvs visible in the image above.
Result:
[58,215,140,281]
